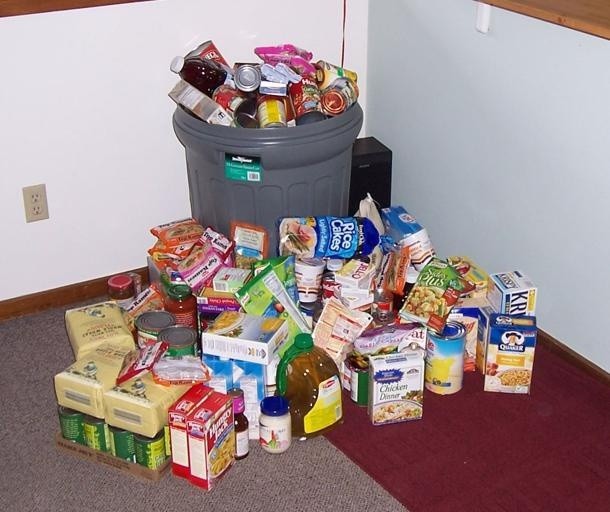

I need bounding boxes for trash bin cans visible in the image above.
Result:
[172,102,362,257]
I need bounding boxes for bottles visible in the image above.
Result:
[321,259,344,309]
[170,56,234,95]
[227,388,250,460]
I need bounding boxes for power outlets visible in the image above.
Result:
[24,183,50,224]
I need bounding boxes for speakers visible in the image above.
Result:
[348,137,392,218]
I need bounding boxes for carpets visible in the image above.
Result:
[314,344,609,510]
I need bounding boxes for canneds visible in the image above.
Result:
[57,403,171,470]
[343,356,369,408]
[133,286,200,358]
[258,395,293,454]
[106,274,137,304]
[376,294,394,313]
[227,387,245,415]
[183,40,359,130]
[425,321,466,396]
